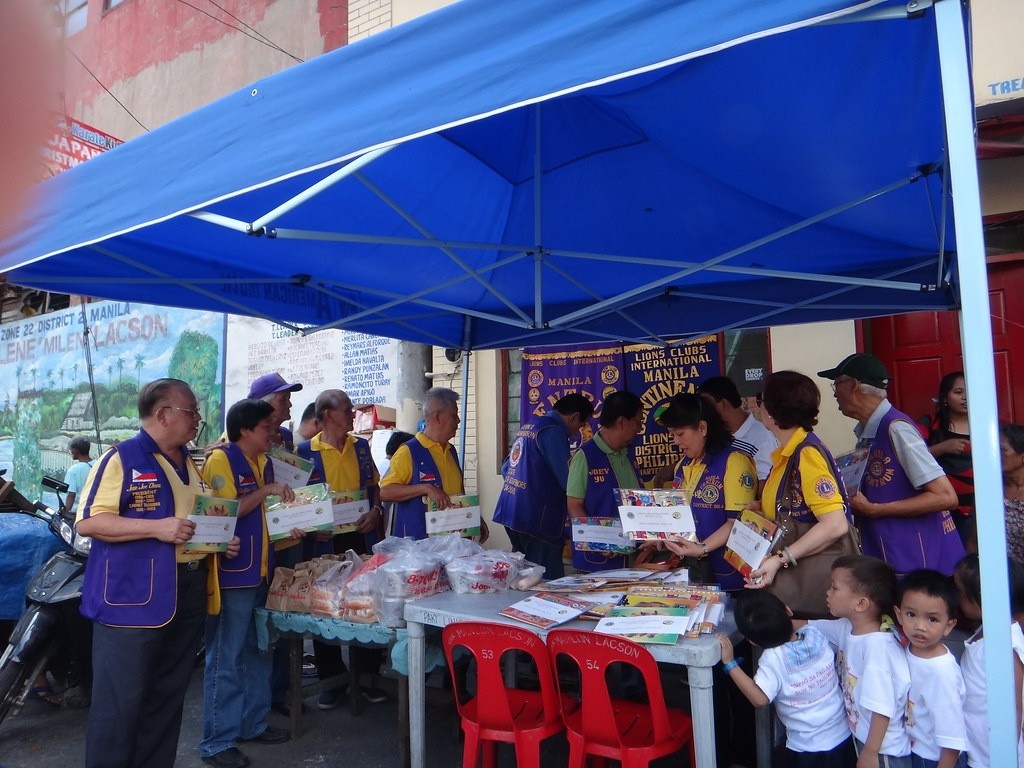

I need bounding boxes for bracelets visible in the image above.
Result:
[777,546,797,568]
[373,504,383,515]
[697,542,708,560]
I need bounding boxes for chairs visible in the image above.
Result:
[545,628,696,768]
[442,621,580,768]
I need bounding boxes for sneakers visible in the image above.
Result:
[300,651,319,677]
[277,690,304,716]
[358,669,389,704]
[200,747,249,768]
[235,724,290,744]
[318,666,351,709]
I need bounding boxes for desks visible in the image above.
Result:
[253,606,471,768]
[405,578,773,768]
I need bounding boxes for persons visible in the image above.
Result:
[790,554,912,768]
[386,432,415,460]
[722,659,739,673]
[999,424,1024,607]
[652,376,778,500]
[714,590,858,768]
[492,393,594,674]
[893,568,970,768]
[379,389,489,705]
[818,354,968,580]
[953,552,1024,768]
[637,394,759,768]
[565,391,645,696]
[731,371,854,768]
[915,371,977,554]
[64,372,386,768]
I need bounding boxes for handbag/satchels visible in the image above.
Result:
[767,446,866,619]
[632,543,686,573]
[312,533,547,629]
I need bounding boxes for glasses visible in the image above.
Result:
[155,407,200,418]
[830,379,859,392]
[755,391,764,408]
[630,419,642,428]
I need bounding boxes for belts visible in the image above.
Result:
[177,559,206,572]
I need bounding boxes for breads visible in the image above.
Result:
[510,573,542,590]
[309,585,378,622]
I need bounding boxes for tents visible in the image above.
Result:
[0,0,1024,768]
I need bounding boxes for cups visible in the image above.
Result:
[378,549,525,629]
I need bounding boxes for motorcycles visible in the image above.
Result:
[0,475,96,732]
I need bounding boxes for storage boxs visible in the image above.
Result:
[352,403,397,433]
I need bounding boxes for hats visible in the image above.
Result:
[819,354,892,389]
[247,372,303,400]
